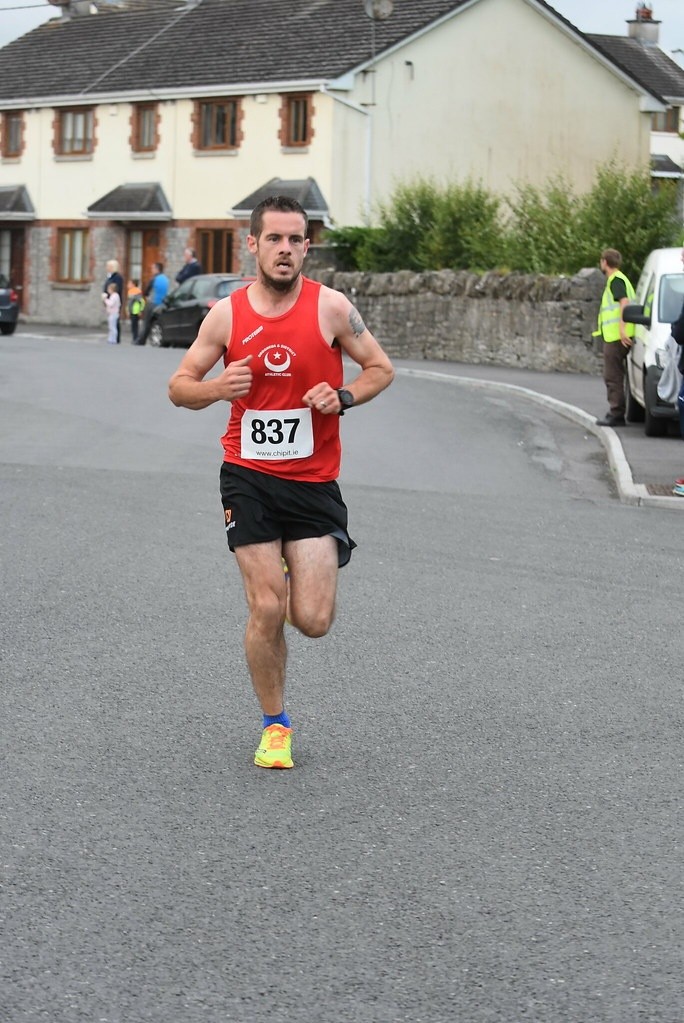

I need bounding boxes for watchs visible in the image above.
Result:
[333,386,354,416]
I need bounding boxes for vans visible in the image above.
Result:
[621,248,683,437]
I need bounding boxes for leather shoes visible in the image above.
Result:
[594,413,627,427]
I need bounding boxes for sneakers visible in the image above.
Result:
[254,723,294,769]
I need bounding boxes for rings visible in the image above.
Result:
[320,401,327,408]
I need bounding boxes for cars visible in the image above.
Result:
[649,129,684,188]
[0,272,23,334]
[147,271,258,347]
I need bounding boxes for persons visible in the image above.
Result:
[102,259,124,343]
[670,247,684,497]
[591,248,639,427]
[101,283,121,344]
[167,195,394,768]
[126,277,145,344]
[133,262,169,346]
[174,246,201,285]
